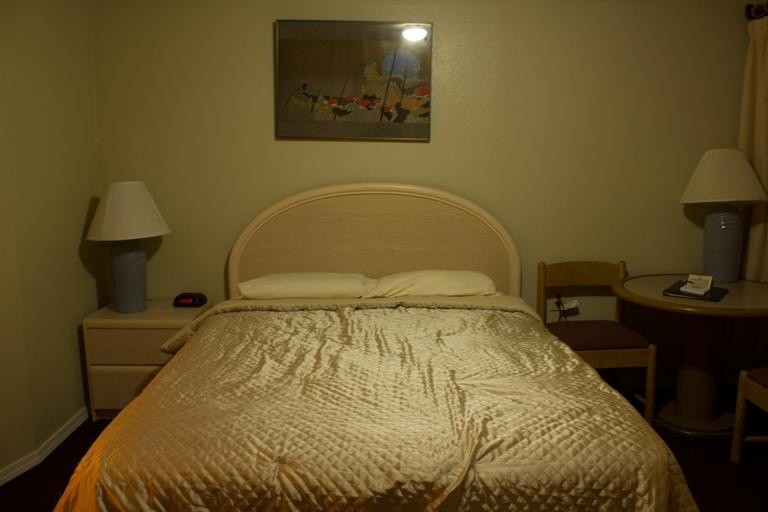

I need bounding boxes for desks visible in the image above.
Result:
[612,273,767,438]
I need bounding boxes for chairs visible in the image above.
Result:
[536,260,657,426]
[731,369,767,464]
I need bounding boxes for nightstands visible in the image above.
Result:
[81,299,197,422]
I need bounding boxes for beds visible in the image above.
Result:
[88,183,672,512]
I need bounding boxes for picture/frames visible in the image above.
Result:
[274,19,434,142]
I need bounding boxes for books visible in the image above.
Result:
[661,280,730,303]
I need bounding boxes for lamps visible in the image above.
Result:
[679,147,767,283]
[83,181,170,312]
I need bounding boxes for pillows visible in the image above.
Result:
[238,269,496,300]
